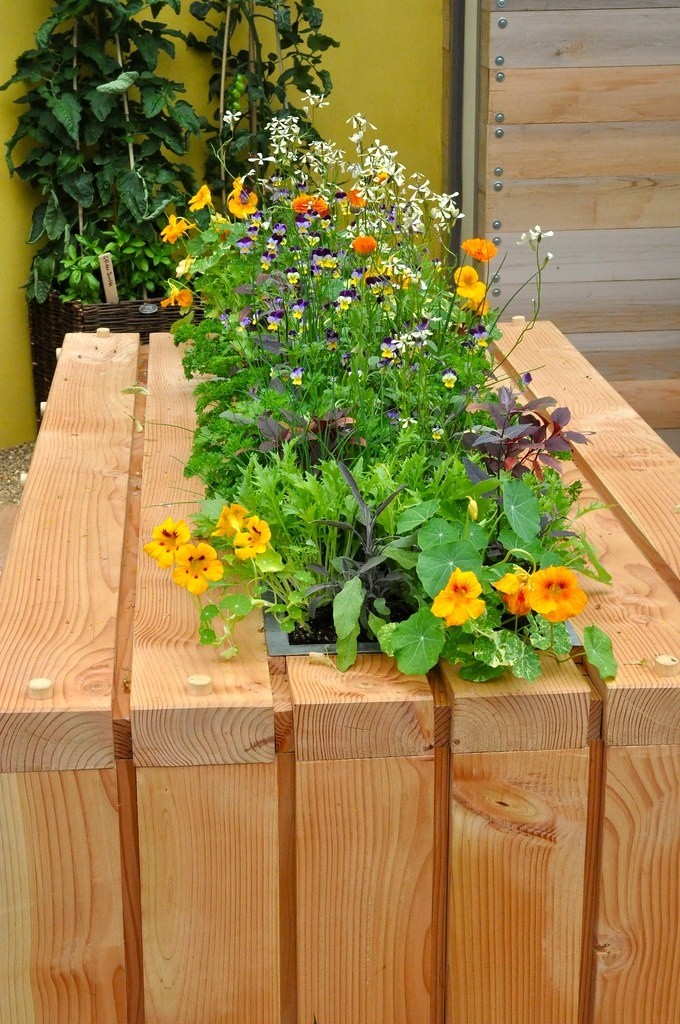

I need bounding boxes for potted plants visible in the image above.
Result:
[1,1,209,431]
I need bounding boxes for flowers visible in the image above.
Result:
[138,88,615,685]
